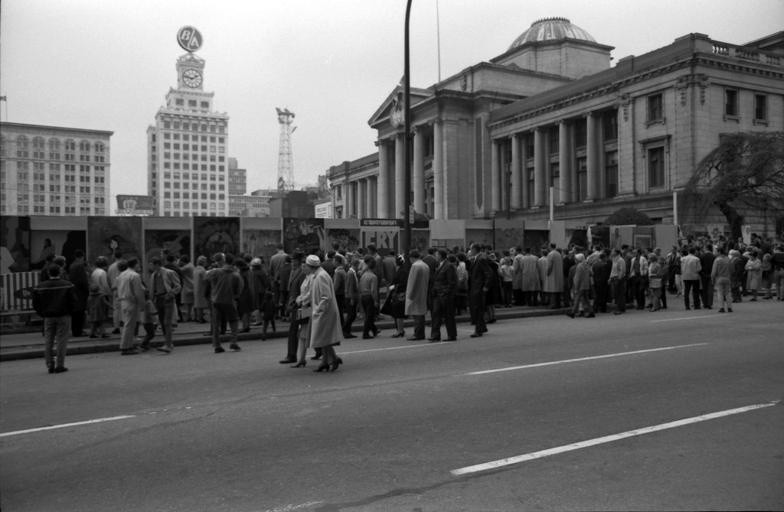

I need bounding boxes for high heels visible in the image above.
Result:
[280,352,342,372]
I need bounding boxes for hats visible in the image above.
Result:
[306,255,321,267]
[575,254,584,261]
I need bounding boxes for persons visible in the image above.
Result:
[2,233,784,375]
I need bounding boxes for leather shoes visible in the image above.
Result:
[344,331,482,341]
[121,346,171,355]
[215,345,241,352]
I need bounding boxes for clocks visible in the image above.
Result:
[183,69,203,89]
